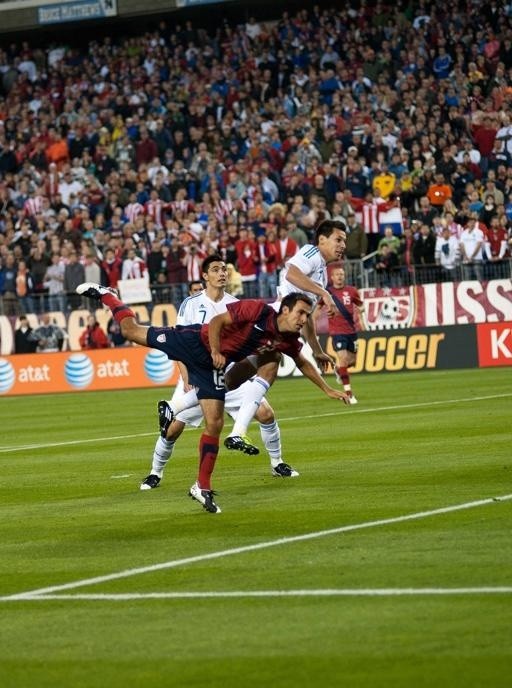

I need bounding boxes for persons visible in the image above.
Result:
[136,254,302,493]
[2,1,511,312]
[14,313,64,353]
[311,265,366,409]
[73,281,351,516]
[155,218,373,456]
[79,315,133,350]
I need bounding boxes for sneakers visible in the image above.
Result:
[224,436,260,455]
[334,366,344,385]
[340,393,358,405]
[75,281,118,300]
[157,399,174,438]
[272,464,300,477]
[188,484,222,514]
[140,473,162,491]
